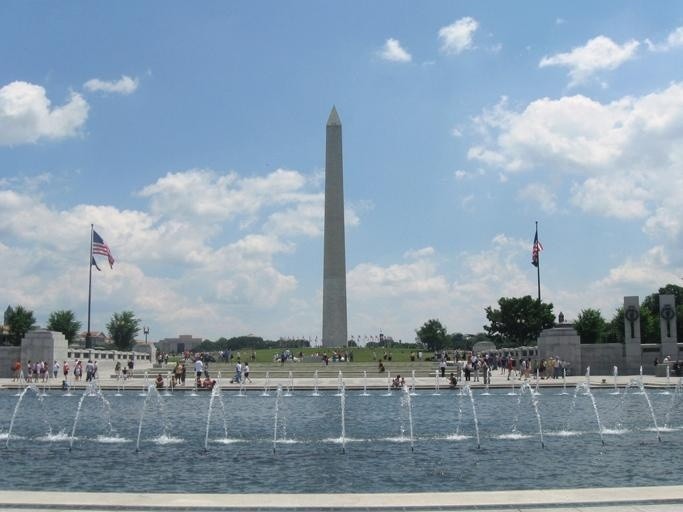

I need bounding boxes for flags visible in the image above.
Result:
[531,232,544,266]
[91,228,114,270]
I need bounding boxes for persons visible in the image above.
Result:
[391,374,406,391]
[115,358,134,381]
[653,356,659,366]
[410,351,568,391]
[155,374,164,388]
[235,360,252,384]
[173,357,216,391]
[663,353,671,364]
[378,359,385,374]
[372,350,392,361]
[157,351,169,365]
[251,349,257,362]
[184,348,240,367]
[272,348,353,369]
[13,358,98,383]
